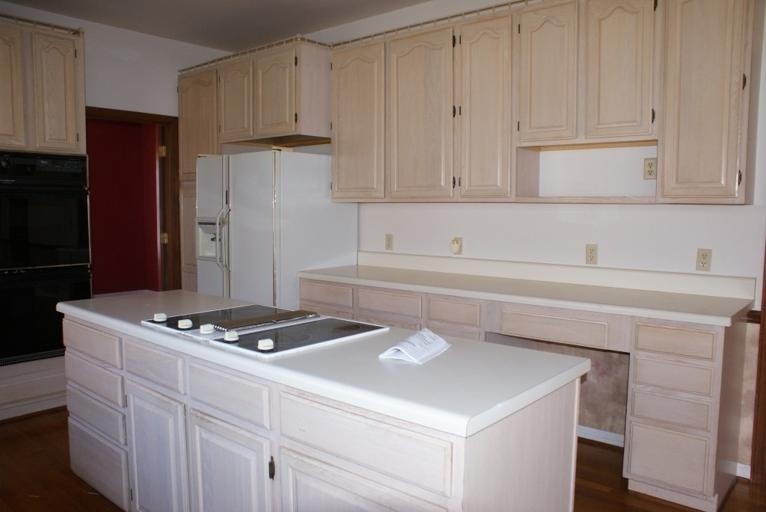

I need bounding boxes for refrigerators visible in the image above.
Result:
[195,150,358,310]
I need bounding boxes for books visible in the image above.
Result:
[377,327,452,365]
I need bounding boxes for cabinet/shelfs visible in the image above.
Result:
[0,14,87,156]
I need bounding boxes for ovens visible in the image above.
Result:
[0,180,93,366]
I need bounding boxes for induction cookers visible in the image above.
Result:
[141,302,387,359]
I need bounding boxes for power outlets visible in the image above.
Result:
[586,244,598,265]
[695,248,712,272]
